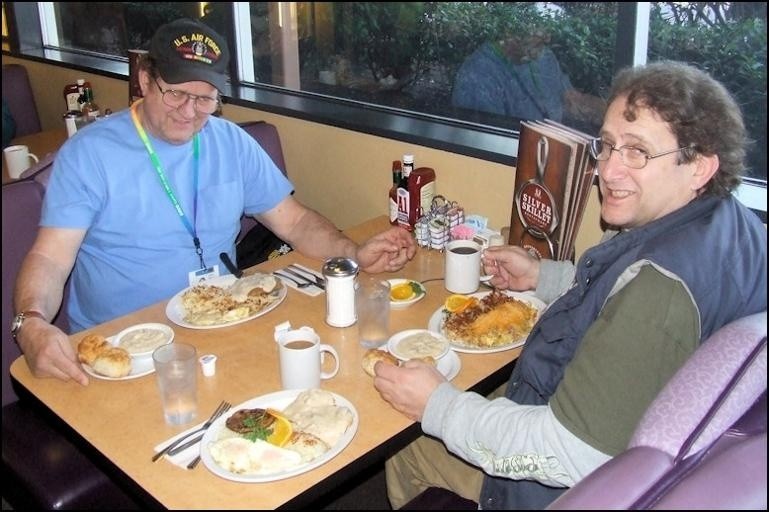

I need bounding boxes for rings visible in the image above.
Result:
[494,259,498,267]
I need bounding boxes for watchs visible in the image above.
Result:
[10,310,46,339]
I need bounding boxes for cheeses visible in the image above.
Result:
[120,327,164,353]
[395,332,447,359]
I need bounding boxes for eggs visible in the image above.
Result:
[248,438,303,474]
[210,437,254,472]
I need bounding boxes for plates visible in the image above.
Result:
[165,273,287,330]
[428,290,548,354]
[114,323,174,357]
[376,344,461,384]
[199,390,359,483]
[386,329,448,361]
[80,334,174,381]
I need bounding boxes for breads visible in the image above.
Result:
[362,346,400,377]
[93,346,134,379]
[77,334,110,365]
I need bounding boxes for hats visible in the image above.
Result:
[144,18,231,97]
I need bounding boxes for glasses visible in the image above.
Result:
[589,136,696,170]
[148,73,223,115]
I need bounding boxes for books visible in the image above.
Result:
[508,118,598,264]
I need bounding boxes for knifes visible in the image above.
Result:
[283,267,325,289]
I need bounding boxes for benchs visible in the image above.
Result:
[0,64,43,138]
[0,124,286,510]
[393,312,769,512]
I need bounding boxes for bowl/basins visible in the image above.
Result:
[382,278,426,310]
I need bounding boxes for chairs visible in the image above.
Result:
[20,150,56,192]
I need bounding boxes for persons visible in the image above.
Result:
[449,4,576,124]
[11,17,418,385]
[372,60,767,511]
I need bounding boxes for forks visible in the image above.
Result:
[152,401,231,460]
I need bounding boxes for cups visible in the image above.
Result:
[151,343,197,426]
[353,274,391,348]
[444,239,498,294]
[278,330,339,389]
[4,145,40,179]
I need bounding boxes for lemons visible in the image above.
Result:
[265,410,293,447]
[445,292,477,314]
[388,284,418,304]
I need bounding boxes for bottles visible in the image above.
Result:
[322,256,360,329]
[397,153,415,232]
[388,160,402,227]
[407,167,436,238]
[62,78,112,138]
